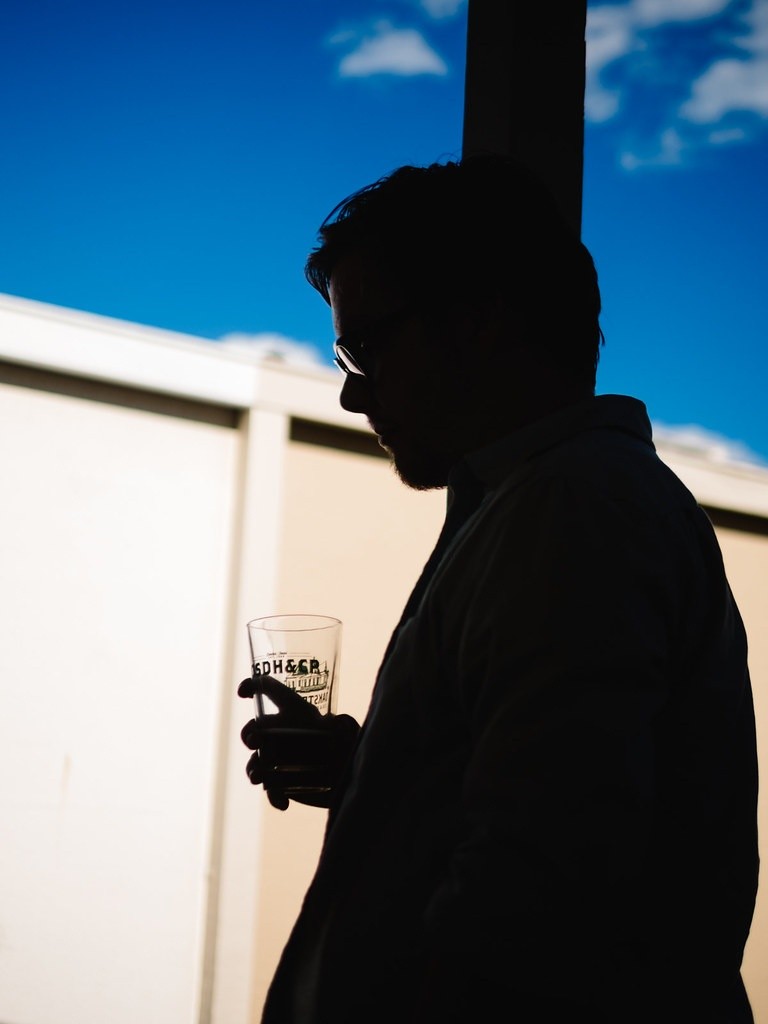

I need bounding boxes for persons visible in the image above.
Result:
[236,162,760,1023]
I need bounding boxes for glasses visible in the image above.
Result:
[331,295,471,378]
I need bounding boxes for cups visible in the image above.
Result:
[245,613,342,793]
